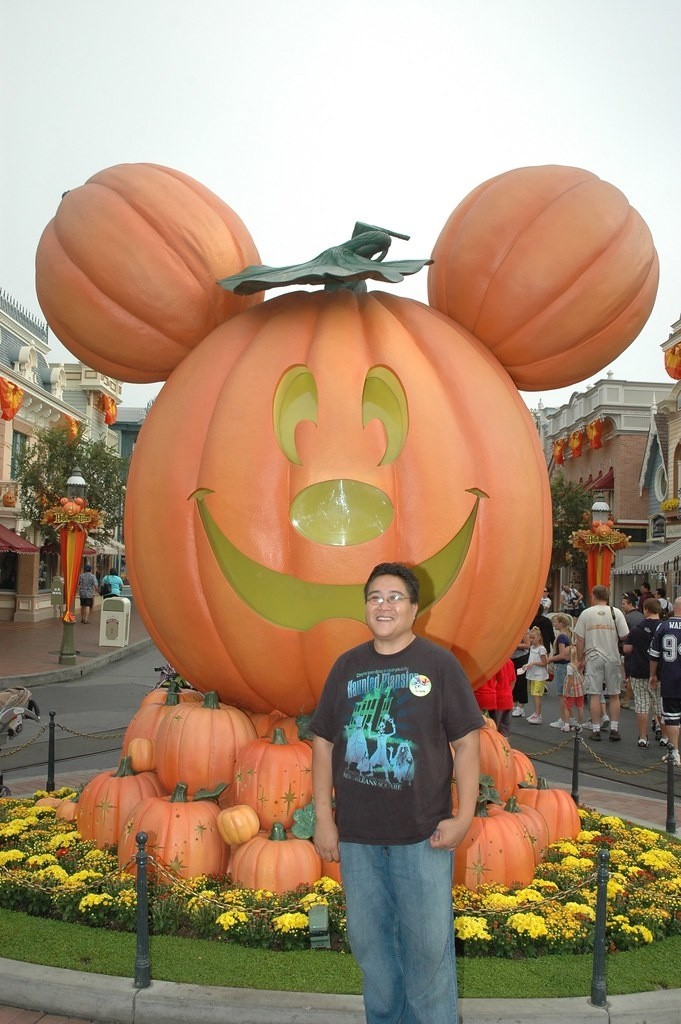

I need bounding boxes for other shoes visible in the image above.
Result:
[81,619,85,622]
[589,731,601,741]
[660,738,669,746]
[638,734,649,749]
[655,729,662,741]
[550,718,576,728]
[582,718,592,730]
[609,728,621,739]
[621,699,630,708]
[561,722,570,732]
[84,620,90,624]
[571,724,583,733]
[600,715,609,728]
[527,712,543,724]
[662,749,680,766]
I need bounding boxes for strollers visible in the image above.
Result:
[0,684,41,797]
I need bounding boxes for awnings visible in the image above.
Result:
[611,539,681,576]
[41,535,125,555]
[0,524,40,554]
[575,469,614,491]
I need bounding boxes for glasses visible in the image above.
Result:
[544,591,547,592]
[366,594,411,605]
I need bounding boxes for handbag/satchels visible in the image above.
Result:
[99,584,112,595]
[579,600,585,609]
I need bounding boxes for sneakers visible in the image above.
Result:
[511,706,525,717]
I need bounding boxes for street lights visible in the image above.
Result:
[60,464,86,664]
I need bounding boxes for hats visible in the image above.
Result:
[86,565,92,570]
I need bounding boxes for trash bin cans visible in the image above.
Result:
[98,597,131,647]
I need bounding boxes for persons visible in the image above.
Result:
[474,583,681,765]
[78,565,99,624]
[308,562,485,1024]
[101,568,123,599]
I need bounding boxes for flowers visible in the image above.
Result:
[568,513,631,555]
[40,498,110,535]
[660,498,679,511]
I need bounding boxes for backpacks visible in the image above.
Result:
[660,601,669,620]
